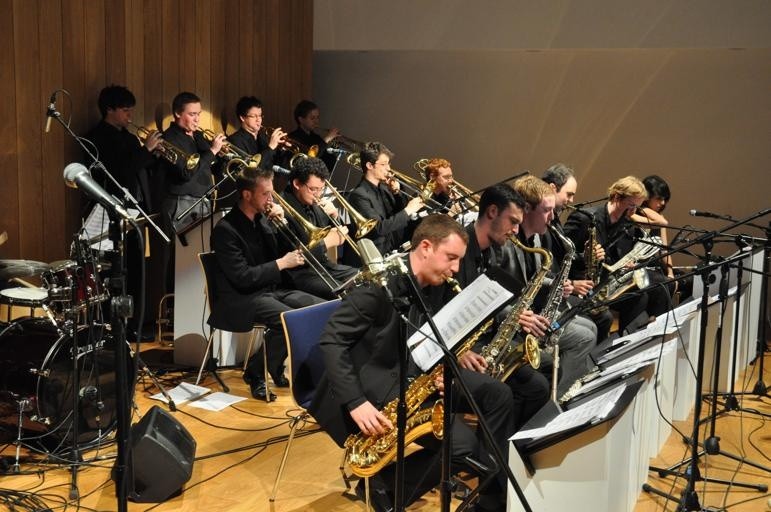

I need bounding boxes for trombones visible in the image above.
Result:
[290,152,378,255]
[226,159,347,301]
[413,157,482,212]
[348,152,458,217]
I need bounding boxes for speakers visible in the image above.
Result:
[112,405,197,502]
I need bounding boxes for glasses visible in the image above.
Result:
[303,183,328,193]
[374,161,393,168]
[244,113,264,120]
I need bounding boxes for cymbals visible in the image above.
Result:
[3,259,46,276]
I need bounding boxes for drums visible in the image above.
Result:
[43,261,78,303]
[1,318,136,447]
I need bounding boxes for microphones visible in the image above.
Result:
[690,210,722,219]
[62,162,134,222]
[42,93,58,134]
[608,208,628,239]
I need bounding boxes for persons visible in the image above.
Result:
[563,176,679,337]
[307,216,514,511]
[205,166,331,402]
[147,92,229,327]
[214,96,292,208]
[81,84,157,342]
[502,173,598,394]
[541,162,614,347]
[450,182,551,438]
[273,156,363,299]
[343,140,425,265]
[401,157,467,255]
[279,100,343,185]
[627,175,694,305]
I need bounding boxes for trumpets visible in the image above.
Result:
[315,126,371,151]
[259,125,319,157]
[127,119,200,170]
[193,126,260,169]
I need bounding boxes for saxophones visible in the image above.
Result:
[566,204,649,316]
[338,274,541,481]
[476,234,552,383]
[602,217,663,273]
[531,222,576,359]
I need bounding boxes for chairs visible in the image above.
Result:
[194,251,273,402]
[269,297,372,512]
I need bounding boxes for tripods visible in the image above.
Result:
[702,258,770,399]
[165,195,231,397]
[659,266,771,478]
[698,242,771,425]
[1,263,120,500]
[112,227,176,411]
[642,228,716,512]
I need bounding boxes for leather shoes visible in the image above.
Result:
[354,475,400,512]
[241,368,278,404]
[267,364,293,390]
[125,328,155,344]
